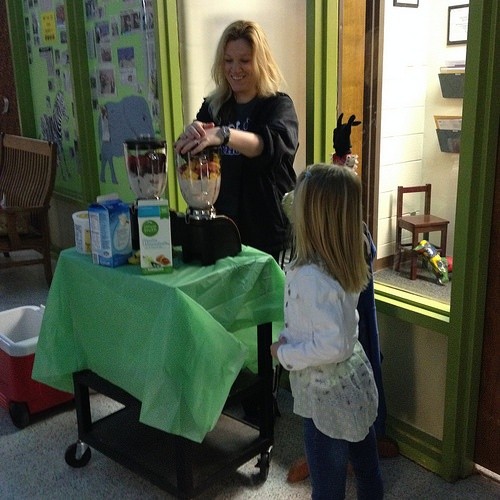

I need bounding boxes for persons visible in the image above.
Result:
[174,19,299,417]
[271,163,385,500]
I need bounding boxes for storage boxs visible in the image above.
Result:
[0,304,76,429]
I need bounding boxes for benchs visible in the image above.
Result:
[0,132,58,290]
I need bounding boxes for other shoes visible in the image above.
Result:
[288,461,309,483]
[377,439,398,457]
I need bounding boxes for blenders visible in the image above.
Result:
[122,134,177,244]
[173,136,242,266]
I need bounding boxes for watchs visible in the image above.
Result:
[218,124,230,147]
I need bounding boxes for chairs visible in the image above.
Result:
[395,183,449,280]
